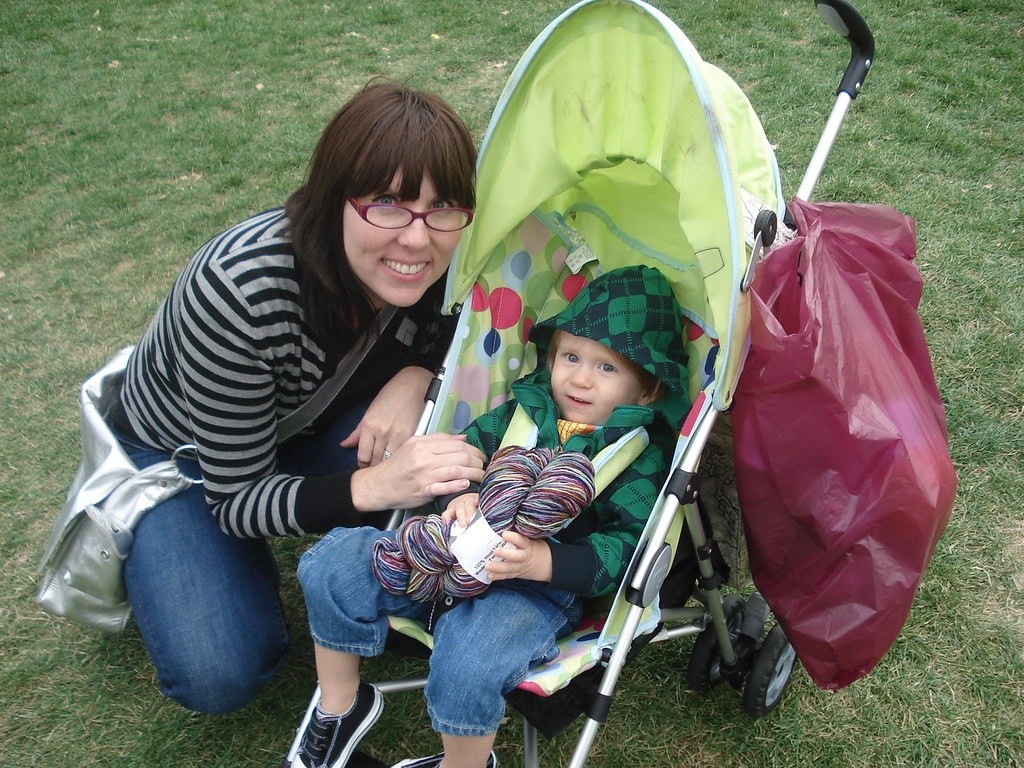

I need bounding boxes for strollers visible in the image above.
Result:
[280,0,880,768]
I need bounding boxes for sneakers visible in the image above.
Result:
[392,748,497,768]
[290,680,385,768]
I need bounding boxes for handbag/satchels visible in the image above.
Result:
[35,346,193,634]
[731,198,958,690]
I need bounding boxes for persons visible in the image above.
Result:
[33,71,486,718]
[286,265,690,768]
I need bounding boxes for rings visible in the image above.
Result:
[383,449,392,460]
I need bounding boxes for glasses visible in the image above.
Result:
[348,194,474,232]
